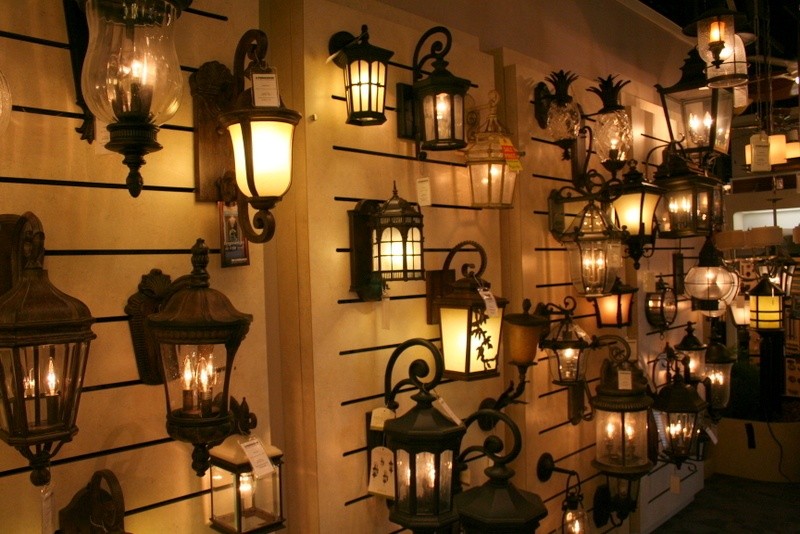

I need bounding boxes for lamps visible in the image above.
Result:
[542,0,800,534]
[0,0,540,534]
